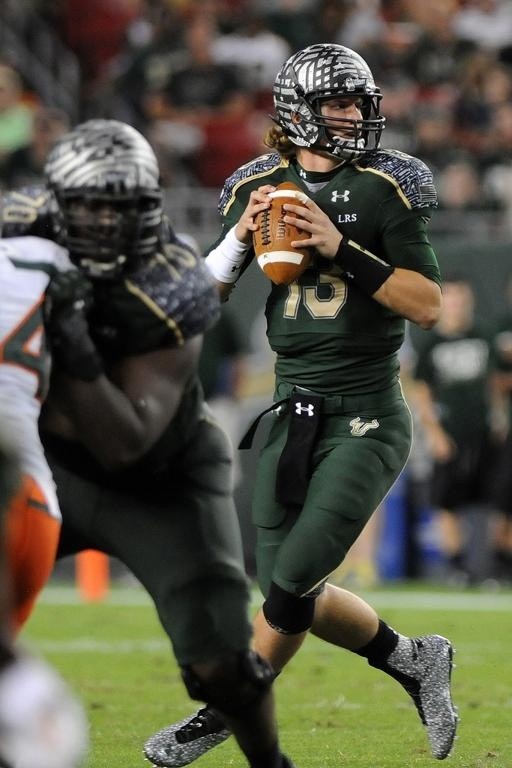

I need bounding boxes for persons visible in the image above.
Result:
[39,116,296,768]
[0,182,91,766]
[143,43,460,768]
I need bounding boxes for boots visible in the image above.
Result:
[145,647,275,765]
[367,625,458,761]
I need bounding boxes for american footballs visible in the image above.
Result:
[252,181,311,286]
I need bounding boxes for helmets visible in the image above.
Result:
[2,184,61,238]
[53,120,161,261]
[273,44,385,161]
